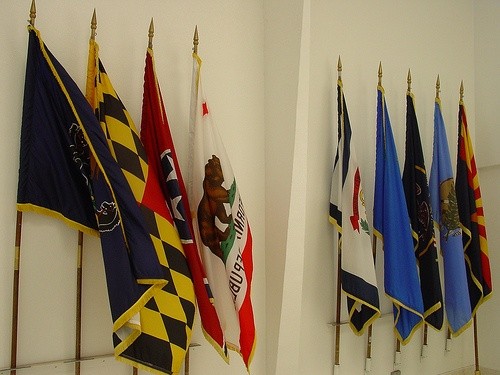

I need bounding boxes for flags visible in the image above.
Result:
[328,78,380,338]
[140,46,231,367]
[454,100,494,318]
[402,91,446,334]
[186,52,258,375]
[429,98,475,340]
[372,86,425,345]
[14,24,197,375]
